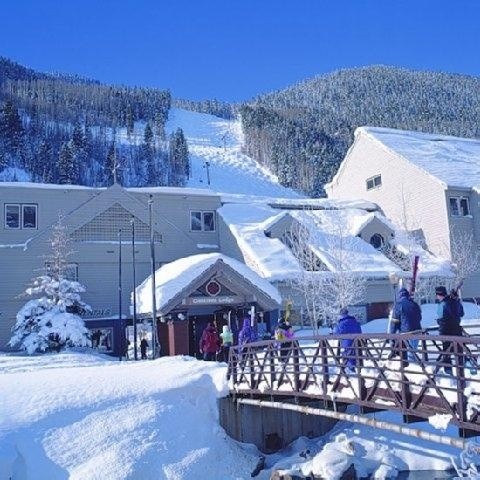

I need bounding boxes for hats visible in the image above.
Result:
[339,308,348,314]
[435,286,447,295]
[399,287,408,298]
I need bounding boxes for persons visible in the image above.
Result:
[125,339,130,359]
[387,308,398,360]
[332,308,364,378]
[390,287,422,373]
[270,317,300,371]
[140,338,149,360]
[198,321,221,361]
[219,324,235,364]
[433,285,465,376]
[238,318,258,371]
